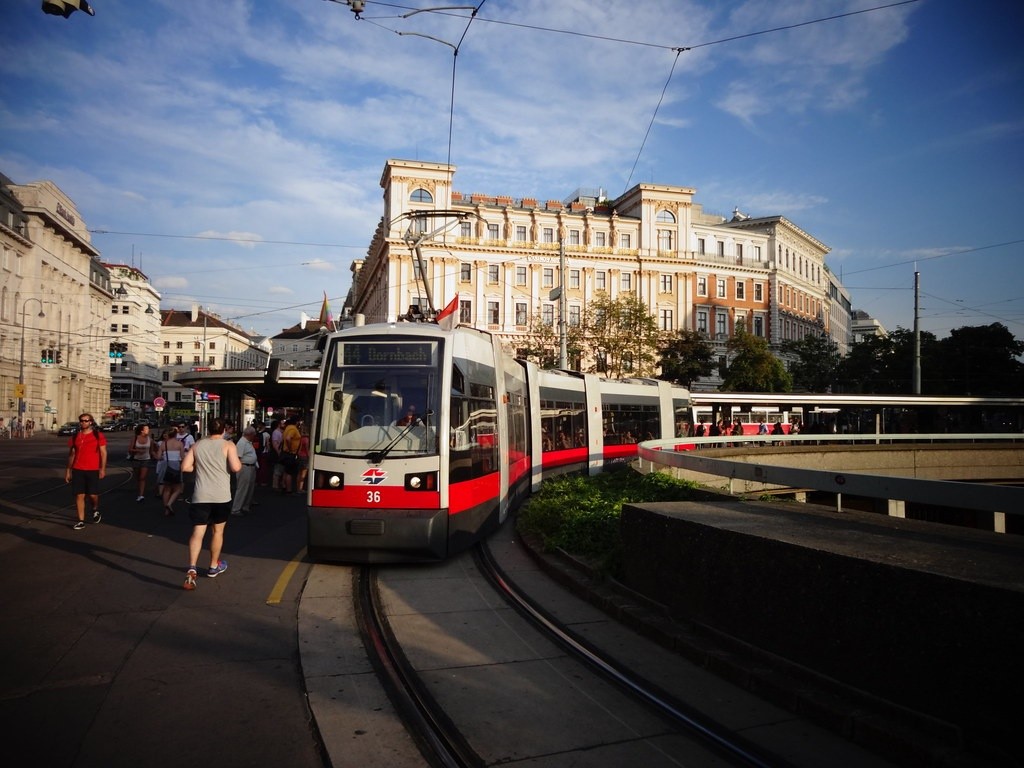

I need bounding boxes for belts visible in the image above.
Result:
[241,463,257,466]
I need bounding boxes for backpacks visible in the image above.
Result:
[253,430,267,454]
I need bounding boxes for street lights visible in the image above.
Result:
[18,298,45,438]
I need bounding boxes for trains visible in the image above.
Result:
[305,209,840,562]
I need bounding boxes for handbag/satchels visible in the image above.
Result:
[267,449,281,466]
[126,450,134,460]
[162,465,181,485]
[279,451,299,467]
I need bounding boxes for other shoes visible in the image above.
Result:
[165,504,174,515]
[73,521,86,530]
[136,495,145,502]
[262,484,306,496]
[232,512,246,517]
[92,509,101,523]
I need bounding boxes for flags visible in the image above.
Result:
[437,296,458,331]
[320,296,331,329]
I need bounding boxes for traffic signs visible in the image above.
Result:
[15,383,26,398]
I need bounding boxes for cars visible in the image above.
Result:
[57,421,81,437]
[99,418,159,433]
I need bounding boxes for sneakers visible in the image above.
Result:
[206,560,228,577]
[183,566,197,589]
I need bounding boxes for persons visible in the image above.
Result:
[231,426,257,517]
[153,420,199,514]
[758,418,769,446]
[129,425,154,502]
[12,416,35,438]
[181,417,243,589]
[270,414,310,493]
[790,419,800,446]
[51,418,58,432]
[711,419,741,448]
[697,419,706,448]
[223,423,235,442]
[542,428,582,451]
[391,403,424,426]
[251,420,270,487]
[66,413,107,529]
[622,432,655,443]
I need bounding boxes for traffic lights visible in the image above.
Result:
[47,349,55,364]
[109,342,129,358]
[41,349,47,364]
[55,350,63,364]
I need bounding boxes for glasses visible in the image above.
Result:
[407,409,415,413]
[178,426,185,428]
[80,419,91,422]
